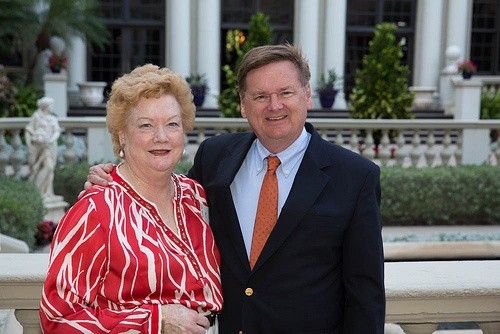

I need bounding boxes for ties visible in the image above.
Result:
[248,156,281,271]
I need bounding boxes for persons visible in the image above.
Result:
[77,41,386,334]
[38,62,225,334]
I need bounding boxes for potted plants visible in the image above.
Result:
[184,70,208,109]
[315,68,339,113]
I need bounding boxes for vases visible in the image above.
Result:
[76,81,107,107]
[462,70,473,79]
[49,65,62,74]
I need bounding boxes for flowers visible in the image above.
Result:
[457,60,476,73]
[44,51,68,70]
